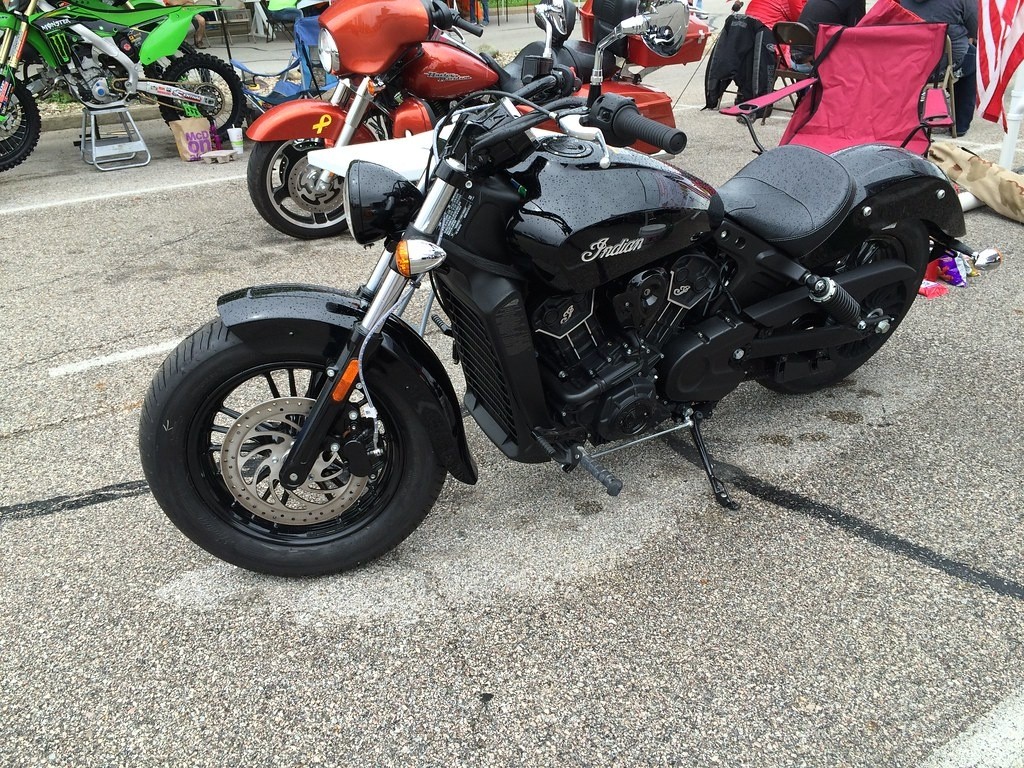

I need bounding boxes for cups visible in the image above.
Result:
[227,128,244,154]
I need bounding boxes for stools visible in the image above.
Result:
[79,105,152,171]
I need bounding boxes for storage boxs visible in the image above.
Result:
[578,0,711,67]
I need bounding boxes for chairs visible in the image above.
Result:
[701,14,778,120]
[760,21,814,125]
[920,32,960,138]
[221,0,257,45]
[198,11,234,47]
[720,20,956,157]
[254,1,295,43]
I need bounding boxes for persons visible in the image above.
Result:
[746,0,808,69]
[168,0,206,49]
[901,0,978,136]
[469,0,490,28]
[790,0,866,92]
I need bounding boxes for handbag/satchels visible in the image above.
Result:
[169,118,213,161]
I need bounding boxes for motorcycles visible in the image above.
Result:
[0,0,248,175]
[137,0,1007,576]
[245,0,715,240]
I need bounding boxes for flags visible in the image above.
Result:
[977,0,1024,134]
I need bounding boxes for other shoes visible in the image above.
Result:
[469,21,477,25]
[949,129,967,137]
[194,34,206,49]
[480,20,488,27]
